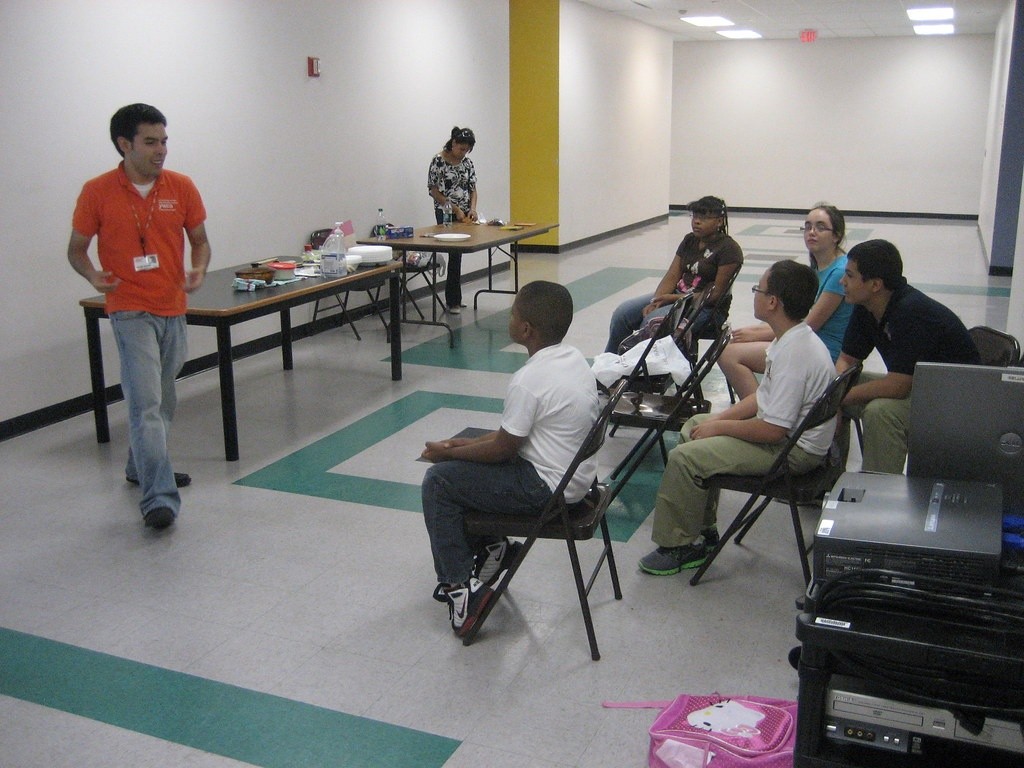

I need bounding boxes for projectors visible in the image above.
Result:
[812,471,1001,602]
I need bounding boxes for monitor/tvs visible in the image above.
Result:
[907,362,1024,518]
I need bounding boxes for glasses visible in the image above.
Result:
[689,213,721,222]
[752,284,785,308]
[800,224,836,233]
[454,131,475,139]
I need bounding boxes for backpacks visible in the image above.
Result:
[647,692,798,768]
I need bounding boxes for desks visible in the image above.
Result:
[79,256,403,461]
[356,221,559,349]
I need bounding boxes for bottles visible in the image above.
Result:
[443,198,453,227]
[376,209,388,241]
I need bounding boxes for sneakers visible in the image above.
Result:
[700,525,720,551]
[433,576,494,636]
[471,536,524,586]
[639,535,708,574]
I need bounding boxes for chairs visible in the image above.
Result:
[463,259,1020,663]
[371,223,446,321]
[310,228,389,341]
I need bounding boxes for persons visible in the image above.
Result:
[428,127,479,313]
[421,281,598,634]
[67,103,212,526]
[776,239,983,507]
[718,207,857,401]
[597,195,743,393]
[639,260,838,574]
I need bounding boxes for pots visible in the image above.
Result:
[235,262,276,285]
[266,259,296,280]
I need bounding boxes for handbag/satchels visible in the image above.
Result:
[394,248,447,276]
[590,335,692,412]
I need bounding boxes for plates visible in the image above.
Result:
[433,233,471,242]
[345,245,393,273]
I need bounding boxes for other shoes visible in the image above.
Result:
[778,488,829,508]
[460,303,467,308]
[446,303,461,313]
[143,506,174,528]
[595,378,642,395]
[126,472,191,487]
